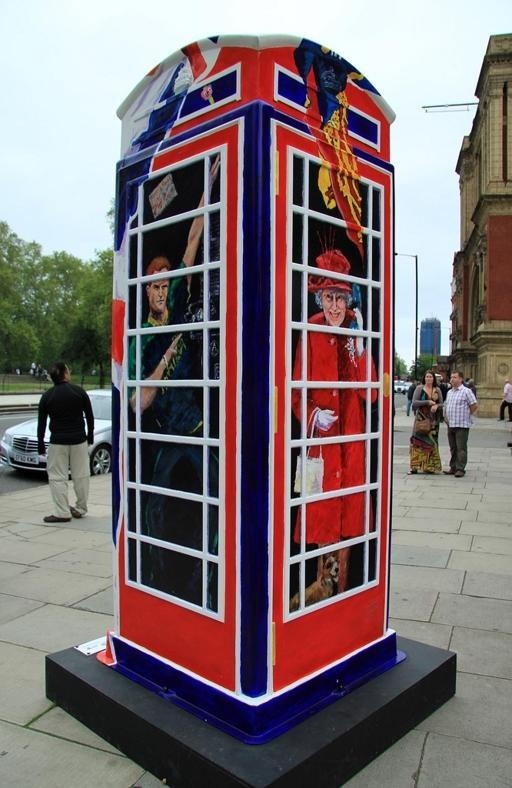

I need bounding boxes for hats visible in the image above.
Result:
[308,249,352,294]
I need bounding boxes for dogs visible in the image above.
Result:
[290,548,342,614]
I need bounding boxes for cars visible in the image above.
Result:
[0,388,112,475]
[393,380,413,395]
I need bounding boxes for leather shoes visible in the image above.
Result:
[44,514,70,522]
[443,467,455,475]
[454,470,464,477]
[69,503,82,518]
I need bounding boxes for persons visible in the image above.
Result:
[498,378,512,421]
[408,370,444,474]
[36,362,95,521]
[127,153,222,611]
[288,250,382,615]
[406,376,476,418]
[443,369,478,476]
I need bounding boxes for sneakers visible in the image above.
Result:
[407,469,418,475]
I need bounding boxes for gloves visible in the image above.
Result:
[314,409,339,432]
[352,308,365,354]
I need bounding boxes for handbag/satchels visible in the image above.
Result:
[414,418,431,433]
[293,454,328,498]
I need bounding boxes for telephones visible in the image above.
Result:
[350,283,362,339]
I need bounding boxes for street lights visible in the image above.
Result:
[395,253,417,378]
